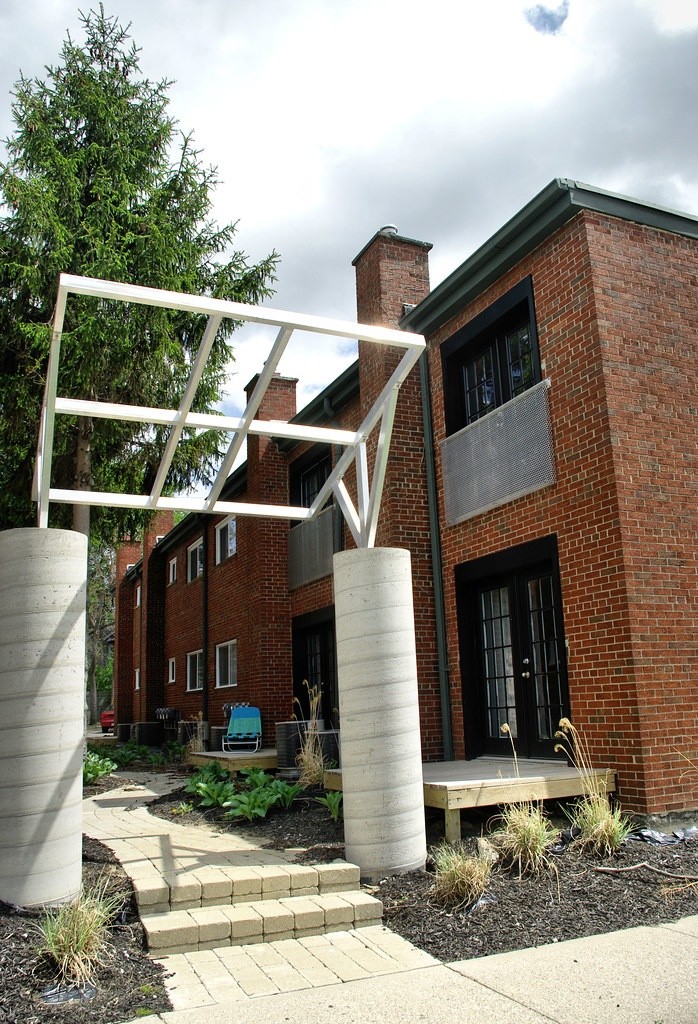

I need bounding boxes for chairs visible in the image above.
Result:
[222,707,263,753]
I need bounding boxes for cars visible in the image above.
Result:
[100,704,114,733]
